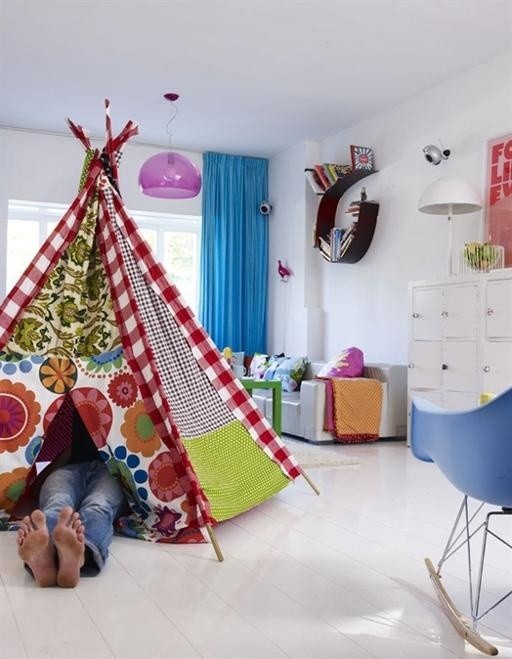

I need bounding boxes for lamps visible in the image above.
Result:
[417,176,483,278]
[136,93,201,201]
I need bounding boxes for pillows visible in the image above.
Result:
[217,346,364,393]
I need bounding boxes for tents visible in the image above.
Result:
[1,96,323,565]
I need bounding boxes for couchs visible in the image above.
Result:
[245,361,406,446]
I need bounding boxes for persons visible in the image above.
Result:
[16,446,127,589]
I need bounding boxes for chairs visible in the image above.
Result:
[409,387,512,659]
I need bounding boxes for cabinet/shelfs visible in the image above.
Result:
[405,268,511,449]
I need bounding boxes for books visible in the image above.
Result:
[304,144,374,262]
